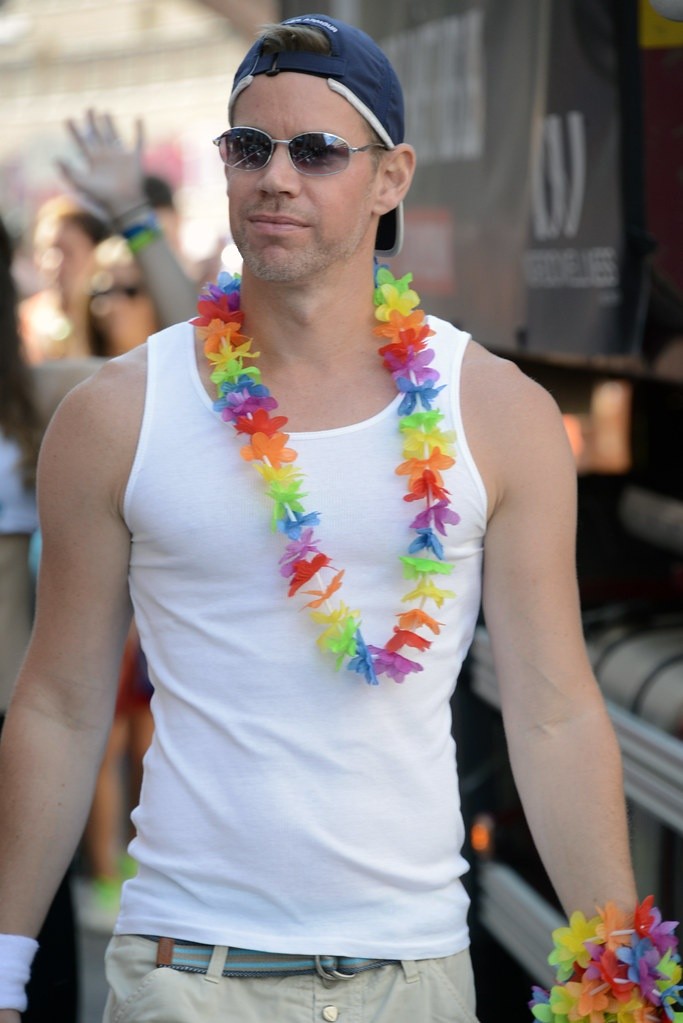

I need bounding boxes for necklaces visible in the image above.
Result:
[191,265,463,685]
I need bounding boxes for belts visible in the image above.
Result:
[139,930,397,980]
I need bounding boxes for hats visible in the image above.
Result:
[228,13,405,257]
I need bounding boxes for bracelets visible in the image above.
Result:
[111,201,162,255]
[1,933,40,1013]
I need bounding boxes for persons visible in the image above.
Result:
[0,14,643,1023]
[1,105,232,1022]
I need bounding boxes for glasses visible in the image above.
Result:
[213,127,388,177]
